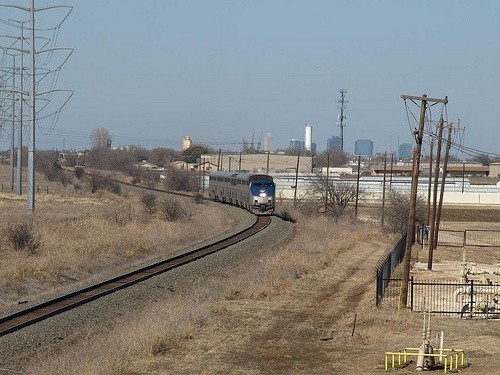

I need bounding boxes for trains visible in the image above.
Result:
[208,171,275,215]
